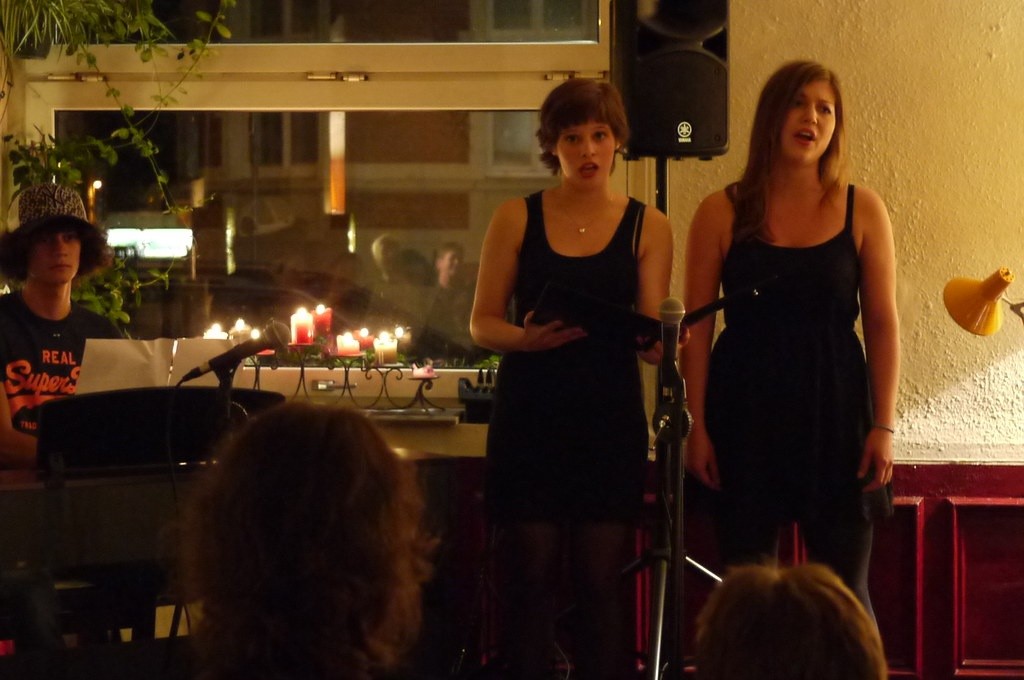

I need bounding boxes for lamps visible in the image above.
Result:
[943,269,1022,334]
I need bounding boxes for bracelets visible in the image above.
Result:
[872,425,894,433]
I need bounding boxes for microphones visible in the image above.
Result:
[660,296,686,401]
[182,322,290,382]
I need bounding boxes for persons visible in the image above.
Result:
[432,241,473,306]
[696,564,887,680]
[469,78,673,680]
[0,184,123,466]
[683,60,901,632]
[160,400,439,679]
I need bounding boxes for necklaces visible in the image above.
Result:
[560,194,613,233]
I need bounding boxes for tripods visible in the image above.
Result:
[619,157,725,680]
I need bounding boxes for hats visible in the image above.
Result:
[6,183,89,233]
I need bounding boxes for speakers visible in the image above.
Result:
[609,0,730,161]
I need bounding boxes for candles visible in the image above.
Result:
[205,313,275,356]
[288,303,314,342]
[311,302,331,338]
[336,320,408,366]
[409,358,439,380]
